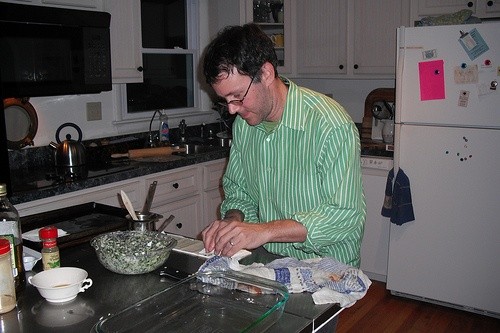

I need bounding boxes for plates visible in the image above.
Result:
[90,266,290,333]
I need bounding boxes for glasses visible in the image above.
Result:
[212,66,261,112]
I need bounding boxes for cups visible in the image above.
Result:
[267,33,284,46]
[126,213,164,232]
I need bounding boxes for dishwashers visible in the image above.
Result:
[358,156,395,282]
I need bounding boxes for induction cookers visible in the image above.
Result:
[28,149,140,188]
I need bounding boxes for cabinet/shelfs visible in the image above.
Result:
[245,0,413,80]
[19,160,227,239]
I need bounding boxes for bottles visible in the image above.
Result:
[0,183,25,315]
[254,2,284,22]
[38,227,61,272]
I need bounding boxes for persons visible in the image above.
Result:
[202,24,367,333]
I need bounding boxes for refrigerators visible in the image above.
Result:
[386,22,500,319]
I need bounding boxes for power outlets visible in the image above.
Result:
[85,101,102,121]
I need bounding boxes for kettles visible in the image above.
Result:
[48,121,89,168]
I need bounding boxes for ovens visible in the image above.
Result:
[0,2,112,97]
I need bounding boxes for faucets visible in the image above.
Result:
[145,109,163,147]
[179,119,213,142]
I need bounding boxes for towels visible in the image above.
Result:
[380,166,416,227]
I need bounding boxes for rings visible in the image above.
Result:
[229,241,234,246]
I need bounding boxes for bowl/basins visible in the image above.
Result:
[28,267,93,305]
[90,230,178,275]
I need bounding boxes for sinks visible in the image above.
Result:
[168,145,221,157]
[186,137,232,147]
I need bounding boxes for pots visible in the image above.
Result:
[2,97,40,149]
